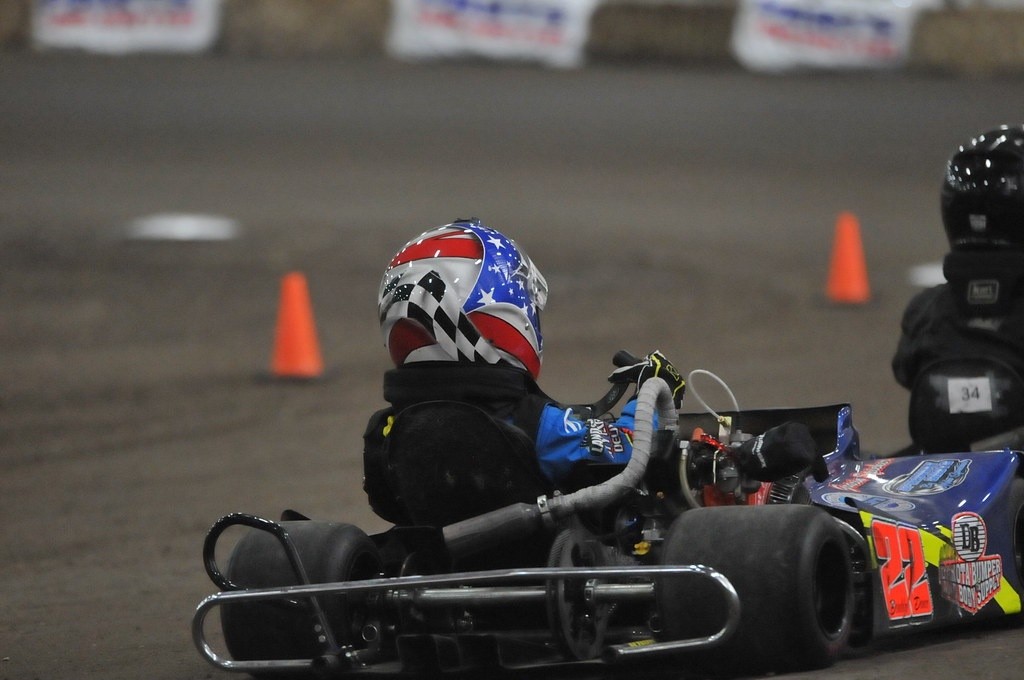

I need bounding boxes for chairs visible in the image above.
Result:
[909,354,1024,455]
[381,401,525,525]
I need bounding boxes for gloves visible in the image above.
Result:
[608,350,683,411]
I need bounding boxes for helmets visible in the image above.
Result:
[940,124,1023,247]
[378,221,542,380]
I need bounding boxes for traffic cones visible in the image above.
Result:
[265,271,327,378]
[821,214,874,306]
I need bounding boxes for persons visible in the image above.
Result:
[361,216,688,532]
[892,124,1024,452]
